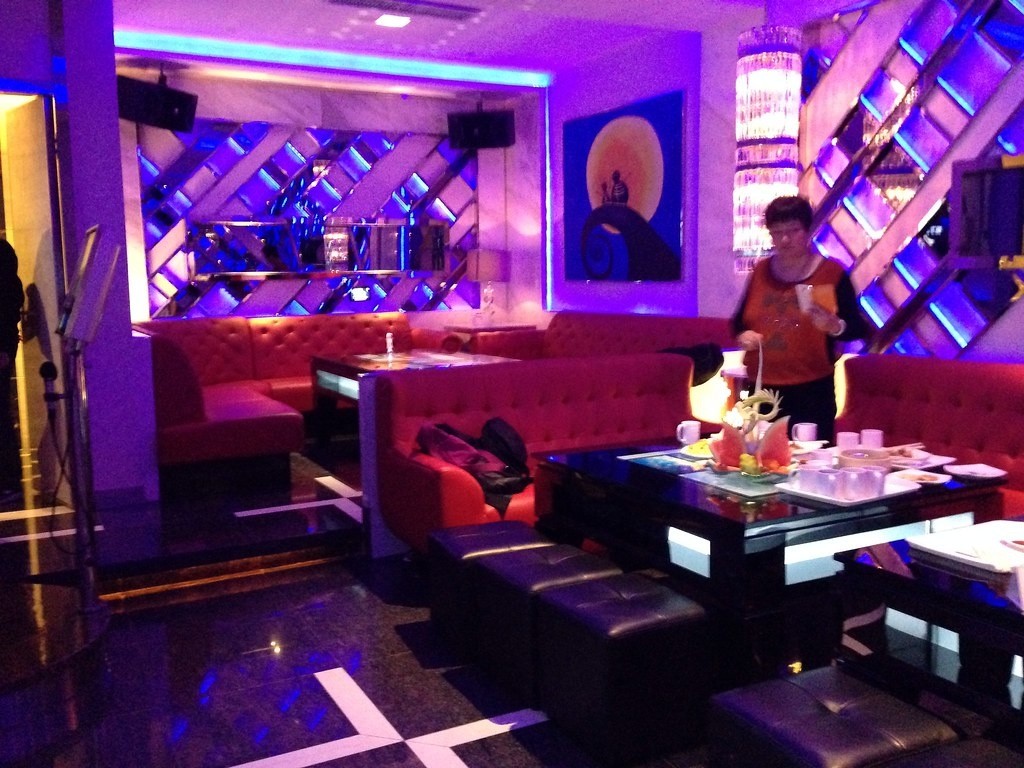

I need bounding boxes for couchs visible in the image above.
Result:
[132,309,1024,565]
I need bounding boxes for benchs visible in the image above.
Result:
[712,655,1024,768]
[427,521,711,758]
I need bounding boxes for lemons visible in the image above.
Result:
[739,452,759,476]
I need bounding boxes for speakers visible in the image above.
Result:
[448,108,516,149]
[117,75,198,134]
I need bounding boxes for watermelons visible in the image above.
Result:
[711,416,792,469]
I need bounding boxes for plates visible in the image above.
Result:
[785,441,823,454]
[884,447,957,470]
[943,462,1008,479]
[680,446,714,459]
[774,473,922,507]
[888,468,951,486]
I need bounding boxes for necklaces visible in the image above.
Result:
[795,252,812,284]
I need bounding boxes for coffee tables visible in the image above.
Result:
[309,348,520,468]
[528,433,1006,682]
[833,515,1024,743]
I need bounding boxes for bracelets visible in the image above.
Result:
[832,319,846,336]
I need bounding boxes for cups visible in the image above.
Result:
[676,421,701,446]
[837,432,859,451]
[792,423,817,441]
[817,469,845,500]
[795,285,814,314]
[810,450,833,468]
[840,468,867,501]
[862,465,888,498]
[789,465,824,494]
[860,429,883,449]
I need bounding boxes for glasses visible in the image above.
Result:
[770,227,804,236]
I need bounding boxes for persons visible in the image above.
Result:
[0,238,24,505]
[729,197,869,448]
